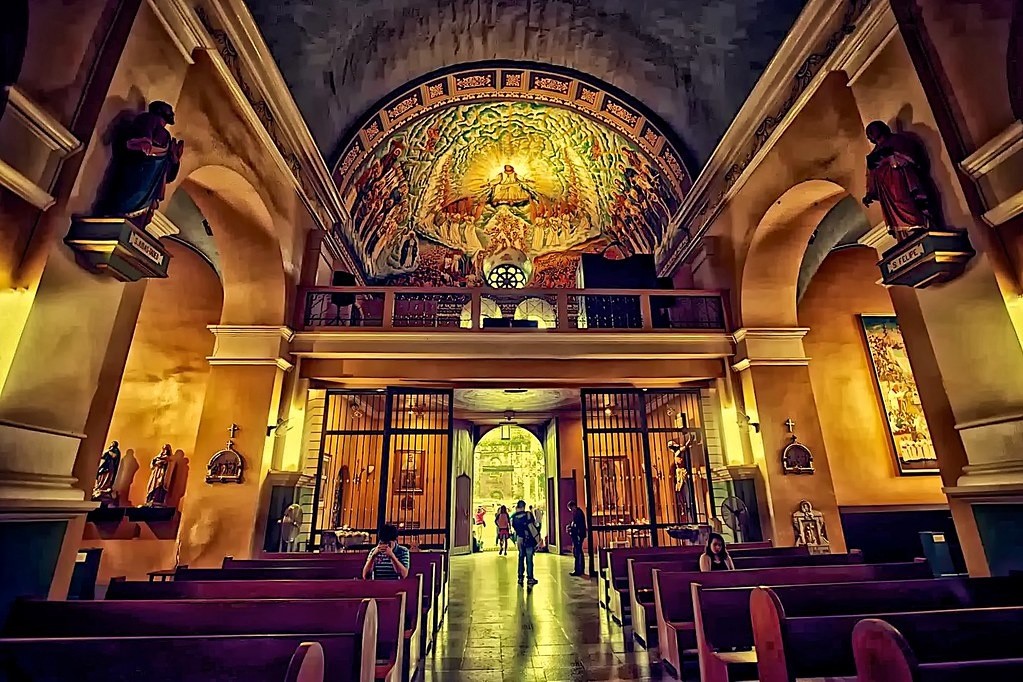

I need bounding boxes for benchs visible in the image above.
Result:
[0,548,450,682]
[387,521,421,544]
[597,544,1023,682]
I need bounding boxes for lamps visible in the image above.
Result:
[606,405,611,414]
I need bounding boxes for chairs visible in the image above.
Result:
[327,271,357,328]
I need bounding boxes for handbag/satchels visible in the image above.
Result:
[483,521,486,527]
[522,511,540,547]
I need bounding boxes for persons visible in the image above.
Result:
[94,440,121,490]
[494,505,544,554]
[362,524,410,579]
[700,533,736,572]
[668,435,694,515]
[92,101,184,229]
[565,500,587,577]
[860,120,943,244]
[511,500,541,585]
[147,443,177,503]
[476,505,486,551]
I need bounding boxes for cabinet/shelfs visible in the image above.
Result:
[576,253,657,329]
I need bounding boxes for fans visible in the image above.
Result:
[267,417,297,438]
[278,503,303,553]
[720,496,750,543]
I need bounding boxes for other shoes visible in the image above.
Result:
[569,570,581,576]
[504,553,507,555]
[518,575,524,584]
[527,576,538,584]
[580,569,584,574]
[499,548,503,555]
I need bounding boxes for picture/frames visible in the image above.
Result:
[860,312,940,477]
[393,450,426,495]
[589,455,629,517]
